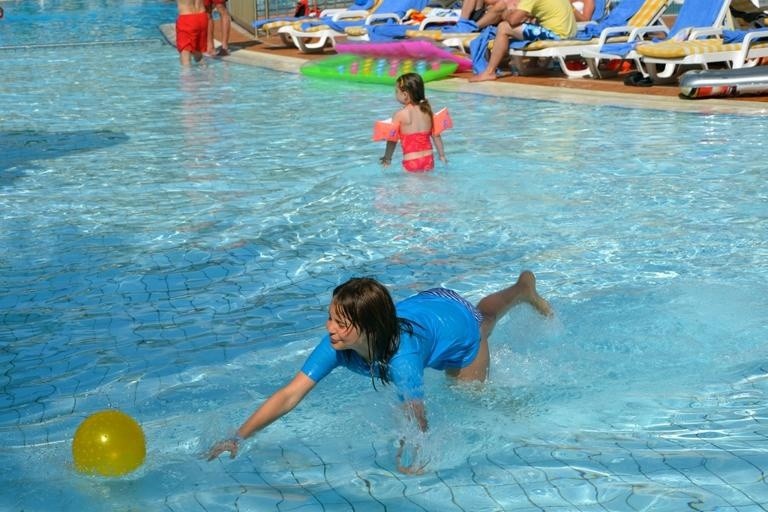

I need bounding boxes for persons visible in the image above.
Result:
[379,72,448,175]
[309,1,320,18]
[172,0,209,66]
[194,270,556,477]
[204,0,231,58]
[293,1,309,17]
[458,0,597,83]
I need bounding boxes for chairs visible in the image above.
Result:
[510,0,672,80]
[633,25,767,80]
[254,0,607,75]
[577,1,732,80]
